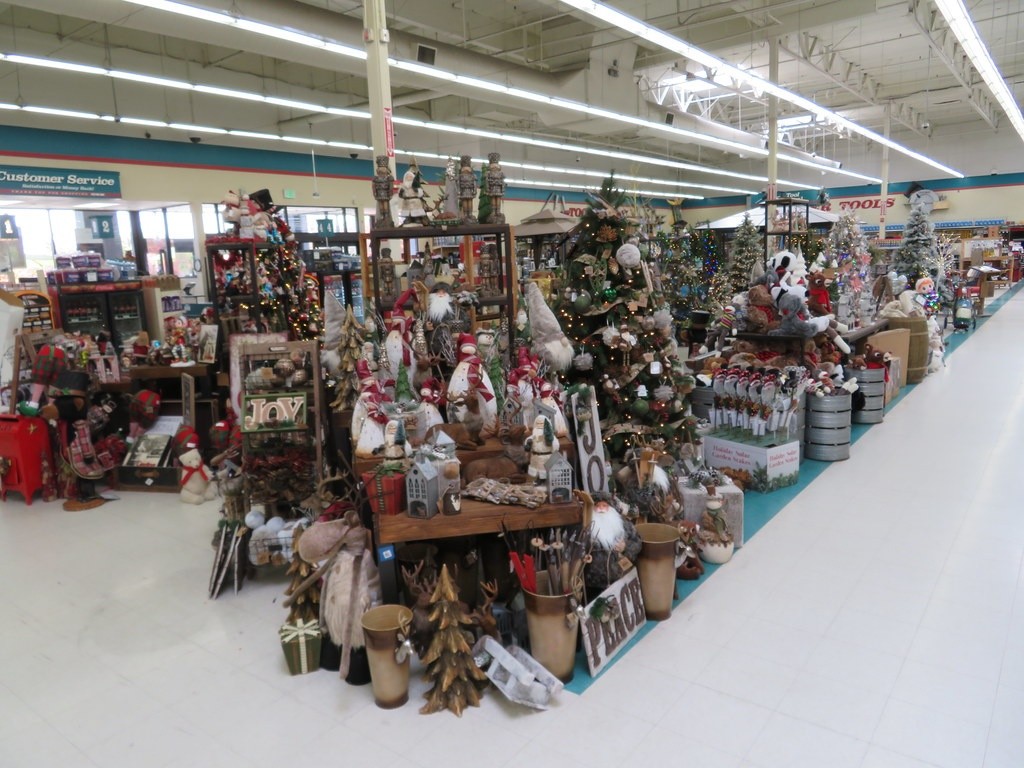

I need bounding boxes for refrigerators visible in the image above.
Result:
[49,282,147,349]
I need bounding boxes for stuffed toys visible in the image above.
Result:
[696,249,859,398]
[178,448,218,505]
[846,343,892,384]
[283,501,382,686]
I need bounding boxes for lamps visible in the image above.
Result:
[0,0,1024,200]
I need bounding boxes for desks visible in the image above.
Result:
[731,317,888,364]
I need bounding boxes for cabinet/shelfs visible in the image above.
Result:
[0,199,1024,605]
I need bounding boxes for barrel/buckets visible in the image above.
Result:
[807,386,851,460]
[845,365,885,424]
[635,523,679,618]
[522,571,583,683]
[362,605,414,708]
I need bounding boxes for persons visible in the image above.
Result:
[586,490,642,600]
[169,314,190,363]
[352,243,570,459]
[371,152,507,228]
[524,414,560,486]
[371,419,414,472]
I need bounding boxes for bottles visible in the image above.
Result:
[325,284,341,297]
[352,282,363,317]
[112,303,137,317]
[106,258,136,281]
[68,302,98,316]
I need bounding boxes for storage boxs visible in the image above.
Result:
[673,476,743,547]
[361,467,405,516]
[279,622,321,676]
[704,430,799,490]
[855,328,910,387]
